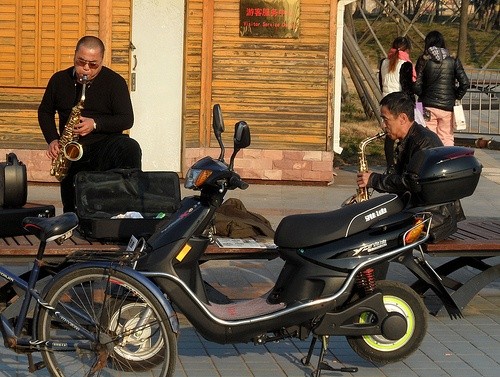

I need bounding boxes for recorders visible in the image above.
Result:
[0,152,27,210]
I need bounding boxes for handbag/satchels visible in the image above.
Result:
[453,98,466,130]
[413,94,426,128]
[209,197,276,239]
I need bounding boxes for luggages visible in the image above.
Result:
[73,166,182,244]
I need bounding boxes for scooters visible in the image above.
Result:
[98,104,485,371]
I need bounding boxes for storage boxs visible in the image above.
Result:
[402,146,483,206]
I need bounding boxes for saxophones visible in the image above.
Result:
[340,131,389,208]
[49,74,89,181]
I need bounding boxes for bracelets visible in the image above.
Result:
[94,122,96,130]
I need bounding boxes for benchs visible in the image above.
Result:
[409,220,500,316]
[0,229,279,305]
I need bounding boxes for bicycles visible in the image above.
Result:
[0,213,180,377]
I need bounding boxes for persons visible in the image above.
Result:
[378,37,417,174]
[357,92,467,245]
[413,30,470,147]
[37,36,142,287]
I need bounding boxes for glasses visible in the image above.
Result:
[75,50,103,69]
